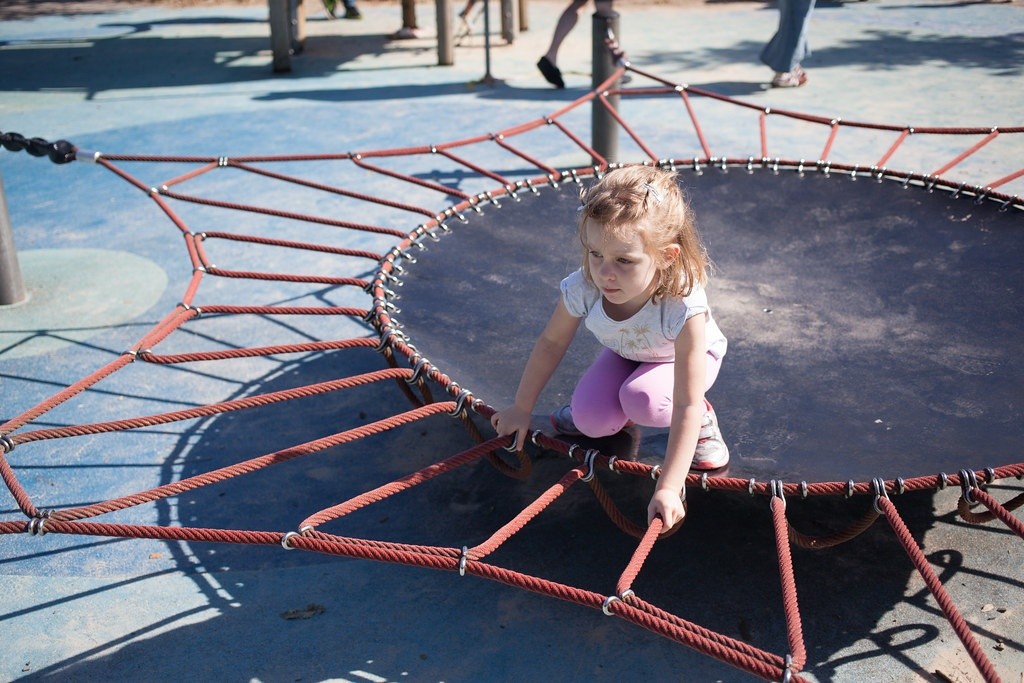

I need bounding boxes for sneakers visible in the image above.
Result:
[691,397,729,469]
[550,404,636,435]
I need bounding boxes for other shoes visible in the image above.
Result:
[622,76,632,83]
[772,65,807,86]
[537,57,564,88]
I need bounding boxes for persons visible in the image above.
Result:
[323,0,418,40]
[759,0,816,87]
[537,0,632,88]
[490,164,731,534]
